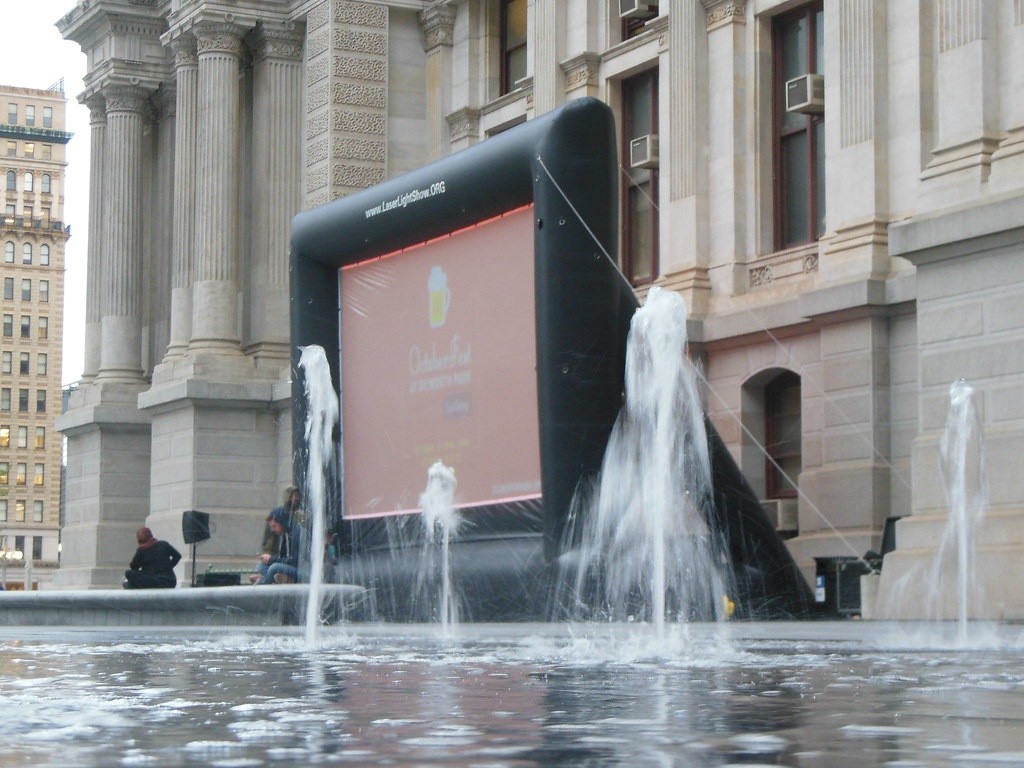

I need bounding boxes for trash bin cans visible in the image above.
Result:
[812,555,863,619]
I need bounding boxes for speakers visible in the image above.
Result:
[182,511,211,544]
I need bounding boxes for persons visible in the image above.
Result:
[248,486,339,584]
[123,525,182,590]
